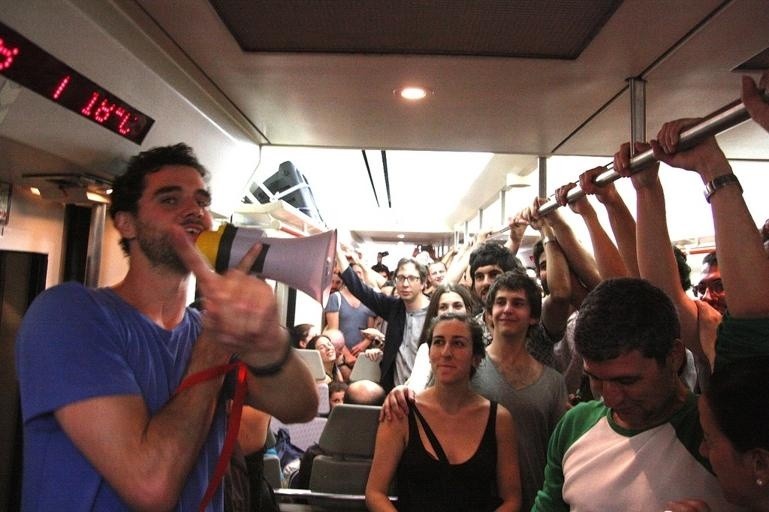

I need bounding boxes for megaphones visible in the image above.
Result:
[195,222,338,310]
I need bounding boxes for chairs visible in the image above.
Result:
[351,350,383,384]
[279,404,399,506]
[291,350,330,416]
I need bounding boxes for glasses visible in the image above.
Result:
[395,274,421,283]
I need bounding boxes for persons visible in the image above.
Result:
[321,262,377,358]
[701,173,743,202]
[614,141,726,392]
[737,69,769,134]
[343,380,387,407]
[327,266,344,295]
[426,235,474,296]
[363,311,523,512]
[520,206,591,343]
[467,237,571,368]
[579,165,692,298]
[291,322,317,350]
[471,272,567,512]
[322,329,345,359]
[652,109,767,318]
[334,244,432,396]
[526,275,728,509]
[239,324,293,379]
[370,264,390,287]
[502,210,528,258]
[302,334,344,386]
[13,142,319,511]
[328,380,347,410]
[541,233,560,251]
[556,182,632,283]
[525,194,602,296]
[661,315,769,509]
